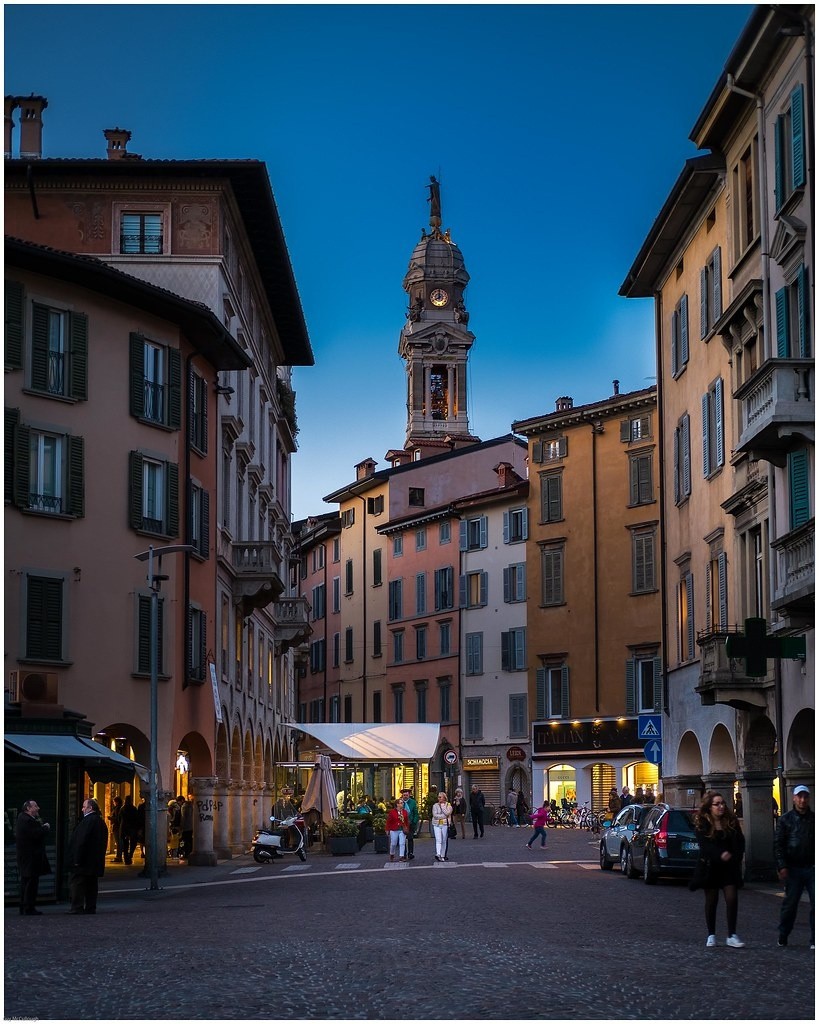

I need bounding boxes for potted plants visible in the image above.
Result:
[324,816,359,856]
[351,812,375,840]
[375,813,391,855]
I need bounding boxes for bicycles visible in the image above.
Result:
[490,801,514,828]
[546,801,608,835]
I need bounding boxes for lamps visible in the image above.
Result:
[549,716,625,724]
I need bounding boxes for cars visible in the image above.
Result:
[599,803,656,876]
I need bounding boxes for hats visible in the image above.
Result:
[794,785,810,794]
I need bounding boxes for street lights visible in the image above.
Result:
[132,543,200,892]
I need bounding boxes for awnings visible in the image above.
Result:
[277,723,441,758]
[78,736,150,784]
[4,735,111,763]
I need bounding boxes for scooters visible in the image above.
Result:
[250,806,307,865]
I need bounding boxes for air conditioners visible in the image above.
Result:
[8,669,59,704]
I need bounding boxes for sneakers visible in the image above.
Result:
[706,935,716,946]
[726,934,746,948]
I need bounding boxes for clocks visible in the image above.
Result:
[430,288,448,306]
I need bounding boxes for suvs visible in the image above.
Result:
[626,803,702,890]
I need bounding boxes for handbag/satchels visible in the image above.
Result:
[403,826,409,834]
[605,812,614,820]
[448,824,456,838]
[524,807,527,813]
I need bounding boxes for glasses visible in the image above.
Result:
[711,802,724,806]
[399,801,404,804]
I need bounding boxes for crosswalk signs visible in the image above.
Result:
[637,714,663,740]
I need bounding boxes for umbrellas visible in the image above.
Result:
[300,755,338,848]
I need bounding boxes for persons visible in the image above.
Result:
[64,798,108,914]
[733,792,743,818]
[384,789,418,862]
[431,783,453,862]
[507,787,530,828]
[170,795,192,861]
[693,791,747,947]
[15,799,50,916]
[424,176,441,217]
[525,801,554,851]
[108,796,145,864]
[772,784,815,950]
[608,785,664,819]
[451,788,467,840]
[469,784,486,840]
[271,786,414,847]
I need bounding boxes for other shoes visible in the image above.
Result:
[435,854,445,862]
[540,845,548,849]
[777,933,787,947]
[526,844,533,850]
[409,853,414,859]
[399,857,406,862]
[389,854,395,861]
[516,824,520,828]
[110,859,122,862]
[473,835,478,839]
[480,833,483,837]
